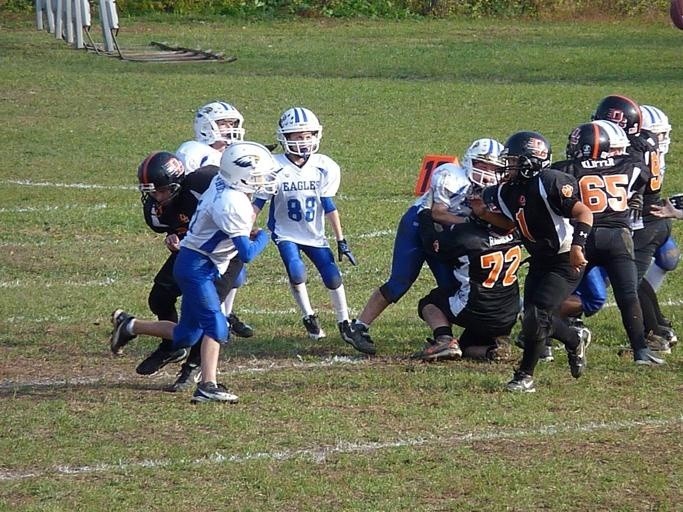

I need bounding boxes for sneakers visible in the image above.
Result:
[413,317,590,392]
[338,319,376,354]
[303,313,326,341]
[110,309,136,356]
[135,344,236,403]
[225,314,254,338]
[633,318,678,366]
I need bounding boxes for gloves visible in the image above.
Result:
[417,187,432,213]
[466,209,492,228]
[660,195,683,209]
[626,193,643,223]
[338,240,355,267]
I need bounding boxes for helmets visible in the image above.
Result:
[567,95,671,160]
[193,102,245,146]
[276,107,322,157]
[138,153,184,216]
[464,132,552,188]
[218,142,282,194]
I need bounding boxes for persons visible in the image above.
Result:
[111,100,357,403]
[339,93,682,393]
